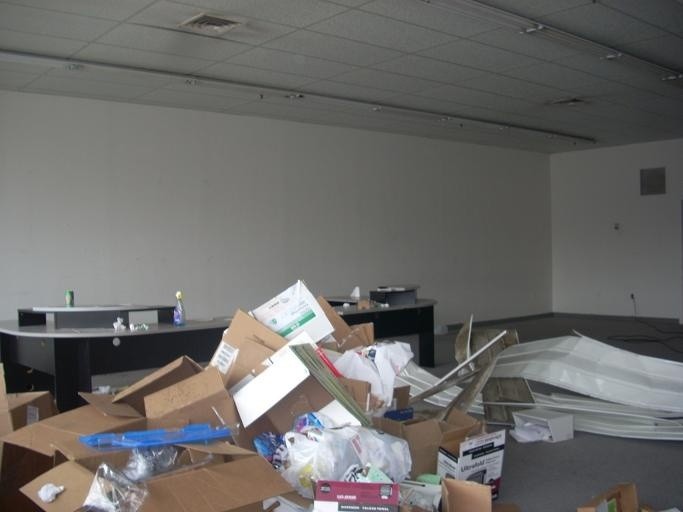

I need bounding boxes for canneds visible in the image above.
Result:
[65,290,73,307]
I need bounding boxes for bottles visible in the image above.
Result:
[171,290,188,326]
